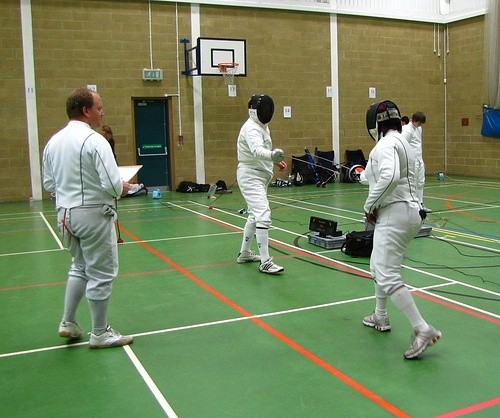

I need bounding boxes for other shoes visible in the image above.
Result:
[422,205,432,213]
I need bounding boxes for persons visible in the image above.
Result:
[401,116,410,125]
[41,88,133,348]
[403,111,431,212]
[359,100,442,358]
[237,94,287,274]
[101,126,116,158]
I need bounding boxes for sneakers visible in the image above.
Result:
[259,257,284,274]
[361,311,392,332]
[404,324,443,359]
[237,250,261,263]
[59,320,84,338]
[89,324,134,348]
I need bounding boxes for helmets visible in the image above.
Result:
[366,100,402,140]
[248,93,275,125]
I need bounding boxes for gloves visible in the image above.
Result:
[353,167,365,182]
[365,210,377,226]
